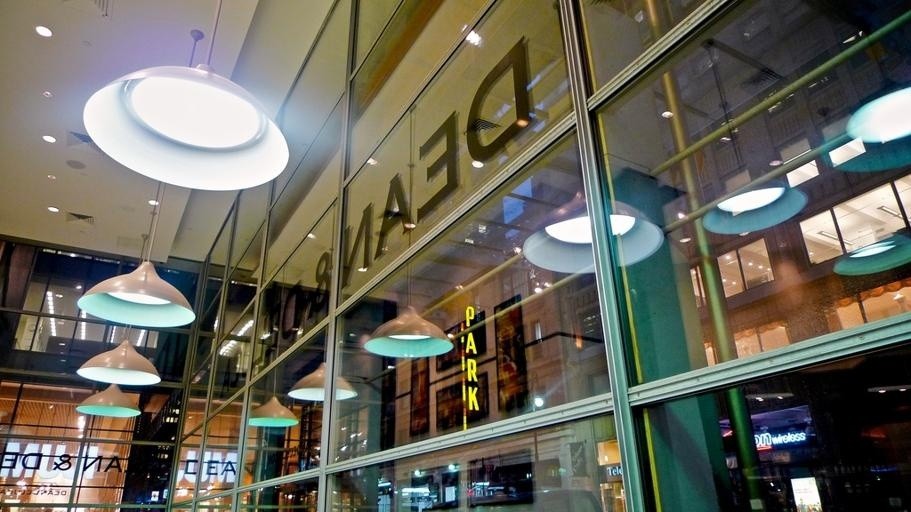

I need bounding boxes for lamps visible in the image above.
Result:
[246,368,300,429]
[288,325,358,402]
[519,149,664,277]
[73,183,197,419]
[365,246,455,360]
[700,46,809,238]
[832,203,911,278]
[813,39,910,174]
[81,28,290,192]
